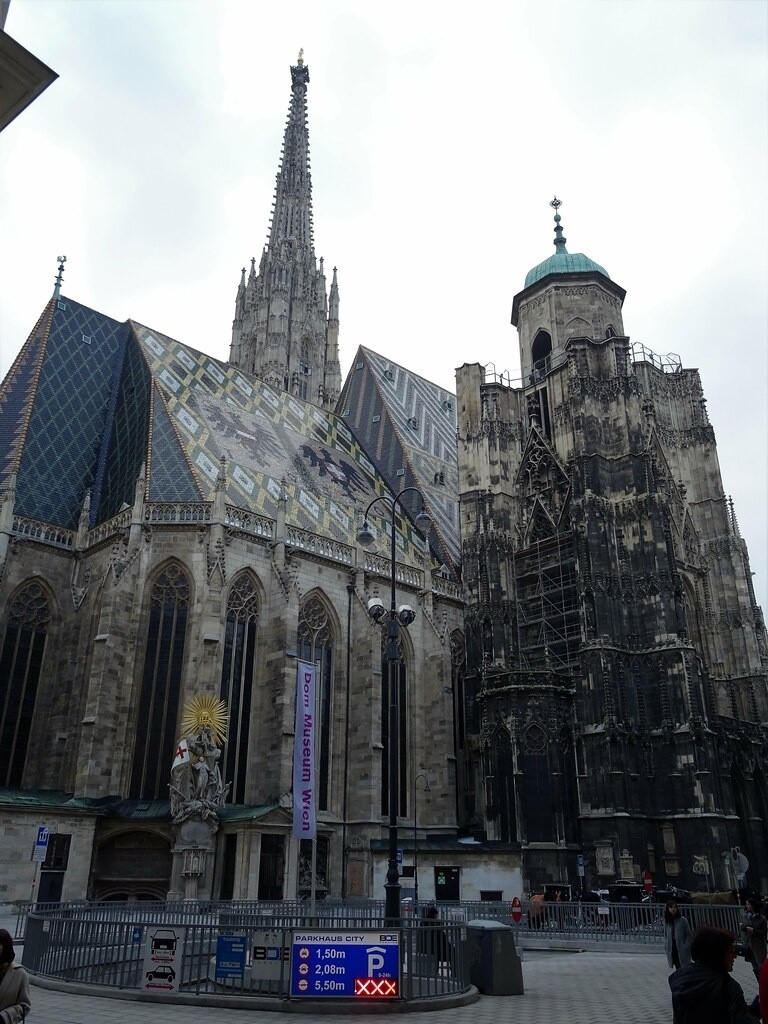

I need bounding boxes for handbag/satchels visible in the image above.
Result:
[732,940,749,956]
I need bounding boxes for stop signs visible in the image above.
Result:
[511,897,522,922]
[642,871,652,892]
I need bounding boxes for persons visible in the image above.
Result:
[664,900,693,971]
[668,926,763,1024]
[416,906,457,977]
[191,756,211,800]
[0,928,31,1024]
[739,897,768,984]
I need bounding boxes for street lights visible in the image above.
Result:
[414,774,431,914]
[355,487,432,926]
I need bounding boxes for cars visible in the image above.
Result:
[654,885,689,899]
